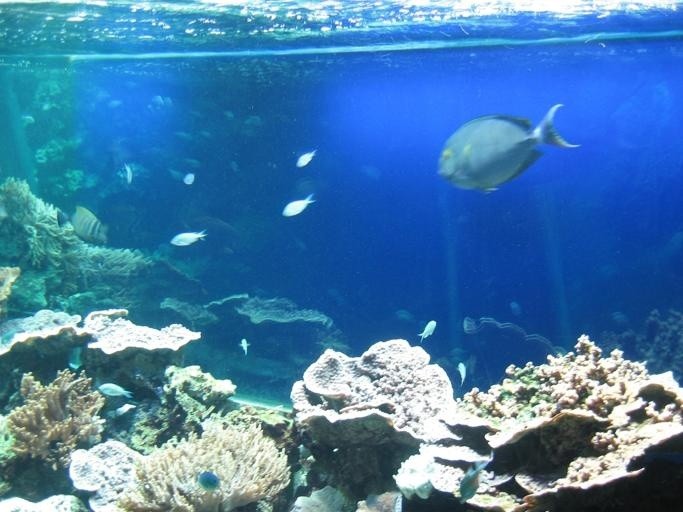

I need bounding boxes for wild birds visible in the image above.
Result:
[454,462,486,503]
